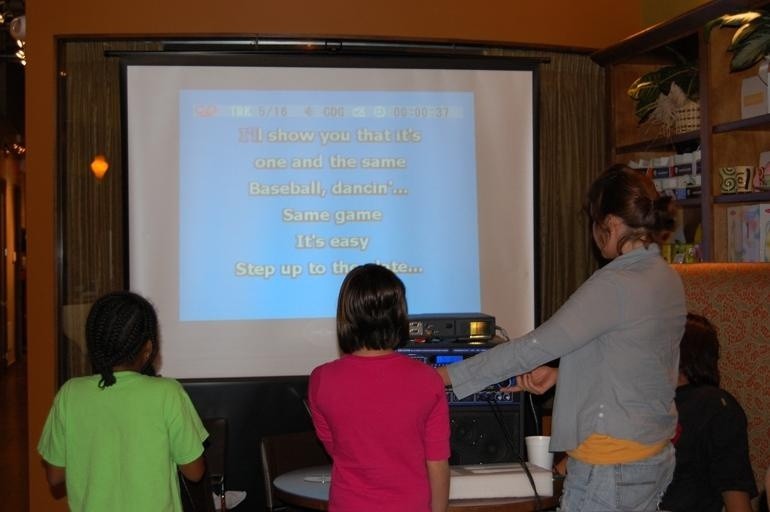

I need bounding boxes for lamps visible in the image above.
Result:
[89,154,109,180]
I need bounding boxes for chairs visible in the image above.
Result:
[258,423,300,511]
[177,417,229,512]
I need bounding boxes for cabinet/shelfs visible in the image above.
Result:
[604,0,770,263]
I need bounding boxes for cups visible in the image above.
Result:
[524,436,554,470]
[719,165,756,193]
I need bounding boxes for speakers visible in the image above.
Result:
[447,405,524,465]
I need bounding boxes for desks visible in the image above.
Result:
[274,461,565,511]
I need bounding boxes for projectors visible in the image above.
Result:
[408,312,496,342]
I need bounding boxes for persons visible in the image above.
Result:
[36,291,210,512]
[435,166,687,511]
[307,264,452,512]
[660,314,758,512]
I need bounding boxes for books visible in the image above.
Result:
[741,73,770,119]
